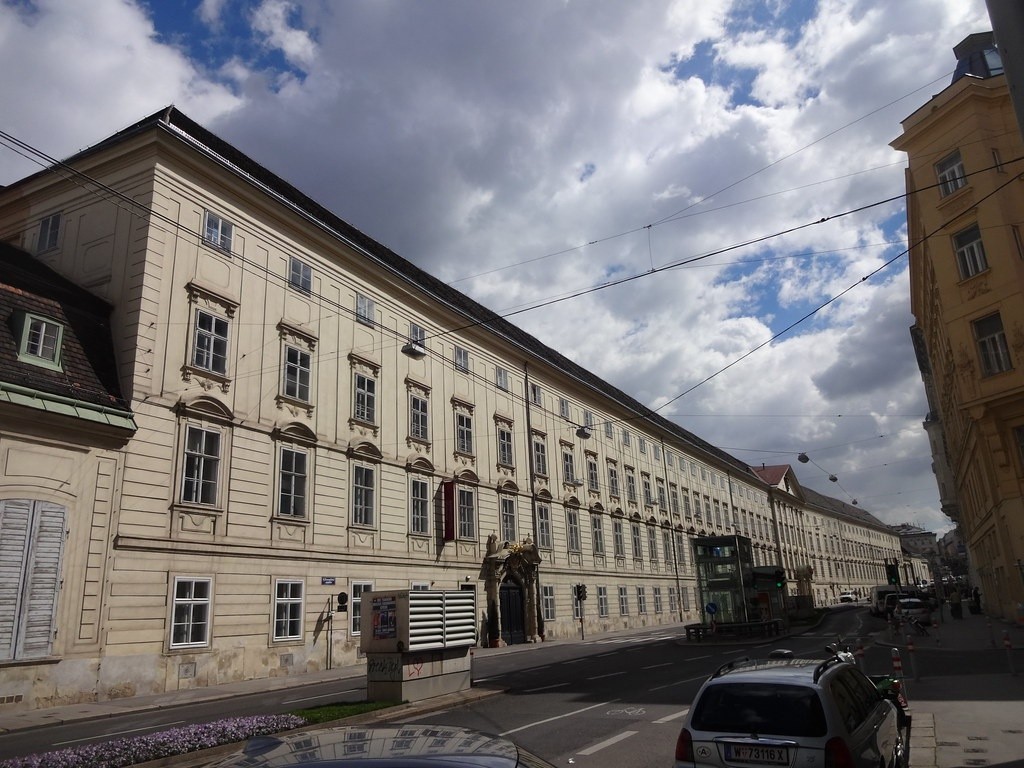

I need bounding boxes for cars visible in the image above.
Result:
[929,579,935,585]
[893,598,930,625]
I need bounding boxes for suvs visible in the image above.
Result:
[673,656,907,768]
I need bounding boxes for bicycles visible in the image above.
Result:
[900,610,930,637]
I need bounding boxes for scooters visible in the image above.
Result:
[863,673,908,728]
[768,633,911,731]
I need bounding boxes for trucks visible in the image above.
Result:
[866,585,896,618]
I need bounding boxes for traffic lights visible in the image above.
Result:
[885,564,899,585]
[774,569,787,591]
[576,583,588,602]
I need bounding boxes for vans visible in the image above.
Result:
[880,593,909,613]
[839,590,859,603]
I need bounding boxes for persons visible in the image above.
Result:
[974,586,983,614]
[949,588,962,618]
